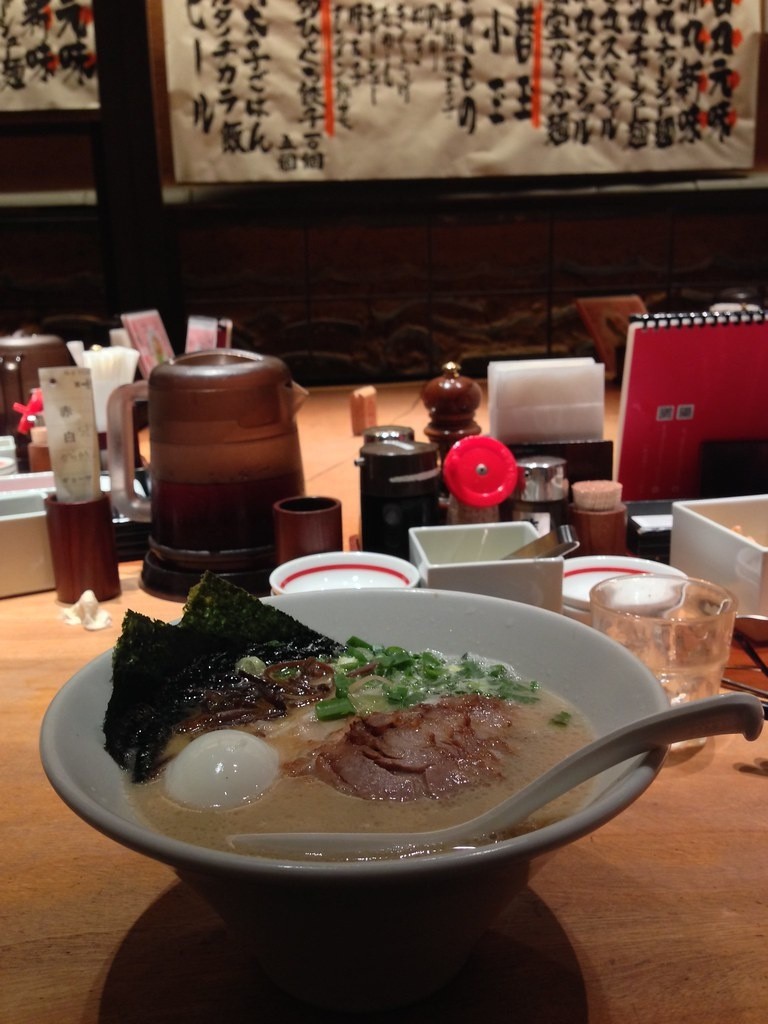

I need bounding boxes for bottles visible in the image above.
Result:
[27,427,51,472]
[358,425,443,563]
[508,457,627,556]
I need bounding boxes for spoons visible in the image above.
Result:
[224,691,763,846]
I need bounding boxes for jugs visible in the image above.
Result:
[0,329,71,473]
[106,349,309,596]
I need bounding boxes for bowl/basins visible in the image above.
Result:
[562,555,689,626]
[669,493,768,617]
[0,470,55,599]
[409,521,565,614]
[38,588,668,915]
[269,551,419,593]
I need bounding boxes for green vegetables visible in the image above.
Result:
[237,634,575,727]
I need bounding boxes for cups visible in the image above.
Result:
[44,492,122,603]
[589,573,736,751]
[274,497,342,565]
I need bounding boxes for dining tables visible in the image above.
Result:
[0,378,768,1024]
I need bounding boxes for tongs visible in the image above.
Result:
[500,524,581,559]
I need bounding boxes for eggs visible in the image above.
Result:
[162,727,280,810]
